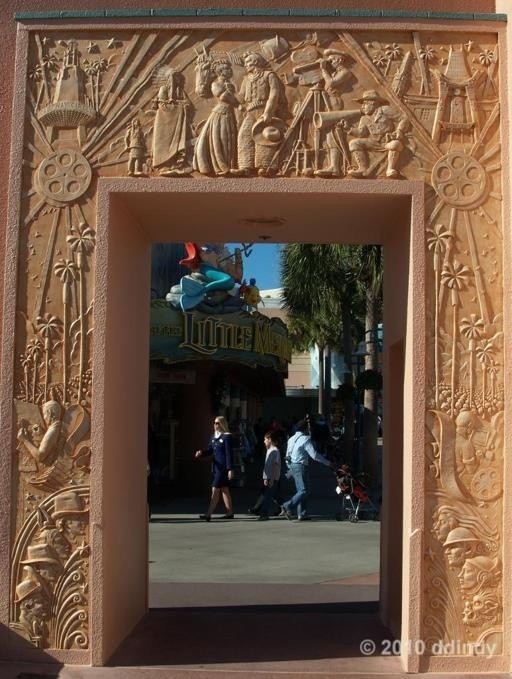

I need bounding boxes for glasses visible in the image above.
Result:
[213,421,220,425]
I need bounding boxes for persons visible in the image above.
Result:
[431,505,500,628]
[246,278,261,313]
[17,400,64,464]
[152,49,409,177]
[341,464,371,503]
[126,118,146,176]
[248,430,282,516]
[7,488,88,625]
[280,420,337,521]
[195,416,235,521]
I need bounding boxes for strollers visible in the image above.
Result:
[333,468,380,523]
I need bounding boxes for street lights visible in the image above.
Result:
[349,338,372,458]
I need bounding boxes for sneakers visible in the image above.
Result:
[198,515,211,521]
[223,512,235,519]
[280,505,293,521]
[297,515,312,522]
